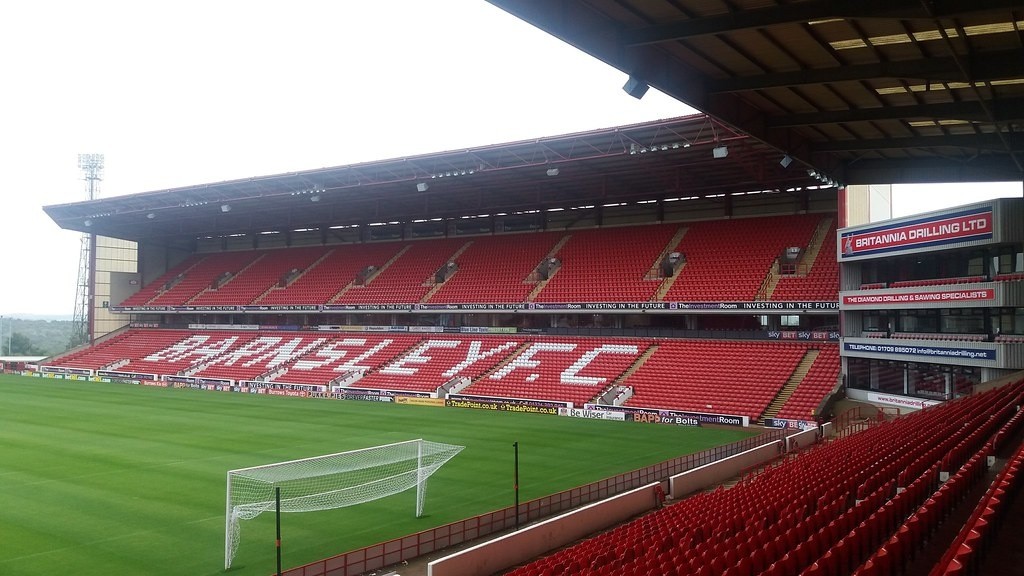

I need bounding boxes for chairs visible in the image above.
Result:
[35,211,1024,576]
